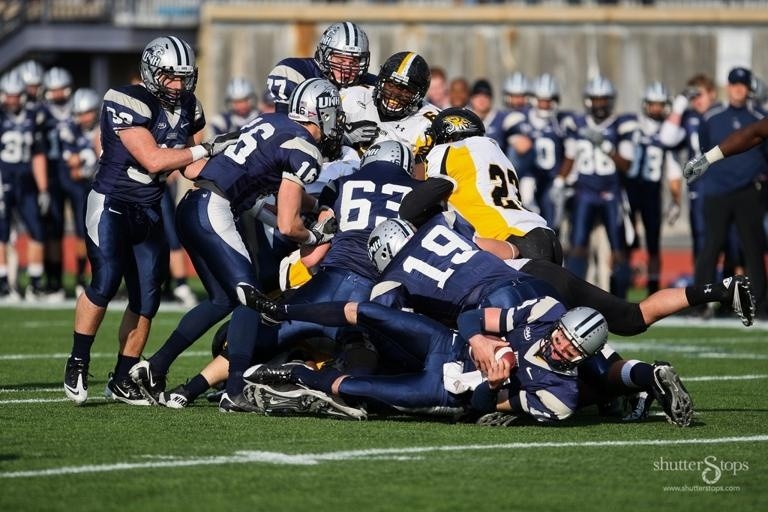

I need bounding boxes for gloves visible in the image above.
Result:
[678,85,703,101]
[36,191,51,216]
[340,120,379,149]
[548,174,568,206]
[200,131,244,161]
[683,153,712,187]
[303,215,336,246]
[665,198,683,227]
[577,126,616,155]
[78,145,100,181]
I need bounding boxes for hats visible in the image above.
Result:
[728,66,756,93]
[469,78,493,98]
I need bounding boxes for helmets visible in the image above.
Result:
[582,75,616,113]
[222,75,261,115]
[427,106,487,147]
[502,69,561,113]
[0,58,72,109]
[640,80,672,118]
[375,49,432,121]
[287,75,344,146]
[315,20,370,90]
[542,306,607,369]
[140,35,199,104]
[359,140,417,177]
[368,218,417,276]
[69,86,103,128]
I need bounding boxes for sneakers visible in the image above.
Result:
[158,386,196,412]
[105,376,149,406]
[0,275,20,304]
[207,389,226,403]
[623,361,694,428]
[175,284,200,311]
[236,282,287,327]
[129,361,165,405]
[24,277,64,306]
[65,355,90,405]
[722,275,757,326]
[73,278,90,300]
[243,383,337,415]
[218,392,265,413]
[241,358,307,385]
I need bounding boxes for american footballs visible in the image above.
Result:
[467,335,516,371]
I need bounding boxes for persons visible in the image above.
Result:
[61,34,213,405]
[0,60,102,321]
